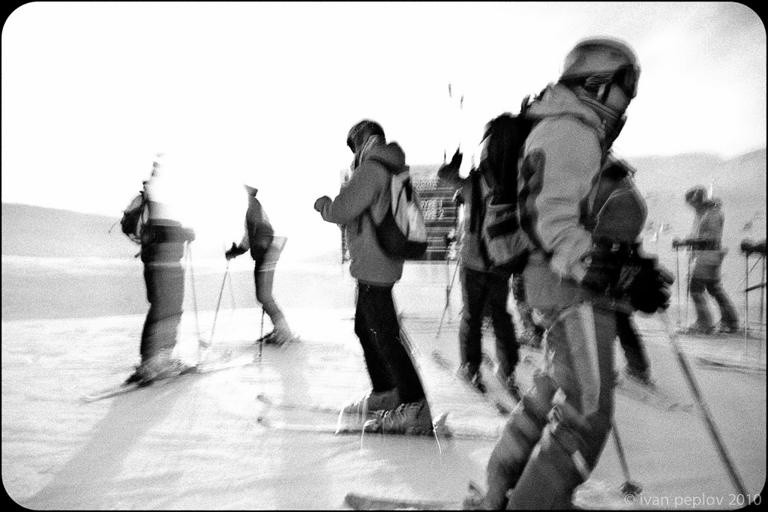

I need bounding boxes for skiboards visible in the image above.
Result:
[253,394,504,439]
[346,492,593,510]
[430,348,524,415]
[612,366,691,410]
[199,336,303,350]
[80,354,254,402]
[642,326,765,339]
[694,354,766,377]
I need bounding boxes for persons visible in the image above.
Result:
[436,148,740,392]
[460,37,676,509]
[225,179,293,346]
[311,120,434,436]
[134,153,196,378]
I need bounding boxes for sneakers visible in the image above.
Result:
[683,322,738,334]
[344,390,398,416]
[517,327,543,351]
[363,402,432,435]
[623,364,651,379]
[258,330,292,346]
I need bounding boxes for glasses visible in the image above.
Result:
[597,65,635,99]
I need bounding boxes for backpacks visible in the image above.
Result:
[472,111,552,274]
[360,154,427,260]
[121,194,148,244]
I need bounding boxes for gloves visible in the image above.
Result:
[226,242,244,259]
[581,247,672,314]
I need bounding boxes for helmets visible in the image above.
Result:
[563,40,635,78]
[346,120,386,151]
[685,186,708,207]
